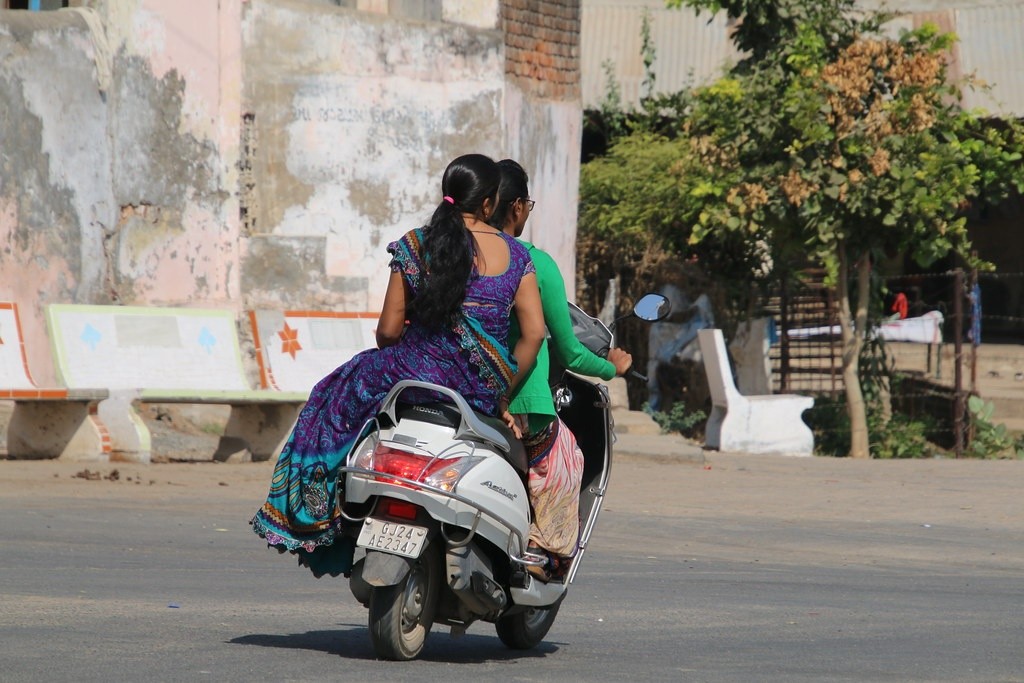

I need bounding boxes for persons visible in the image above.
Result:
[249,152,632,584]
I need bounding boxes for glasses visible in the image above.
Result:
[511,198,535,211]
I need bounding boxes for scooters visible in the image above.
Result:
[336,293,671,661]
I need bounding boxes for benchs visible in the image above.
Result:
[248,311,381,392]
[45,303,311,464]
[0,301,114,462]
[697,328,816,456]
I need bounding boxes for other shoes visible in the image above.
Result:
[527,565,552,583]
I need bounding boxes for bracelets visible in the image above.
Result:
[502,396,509,402]
